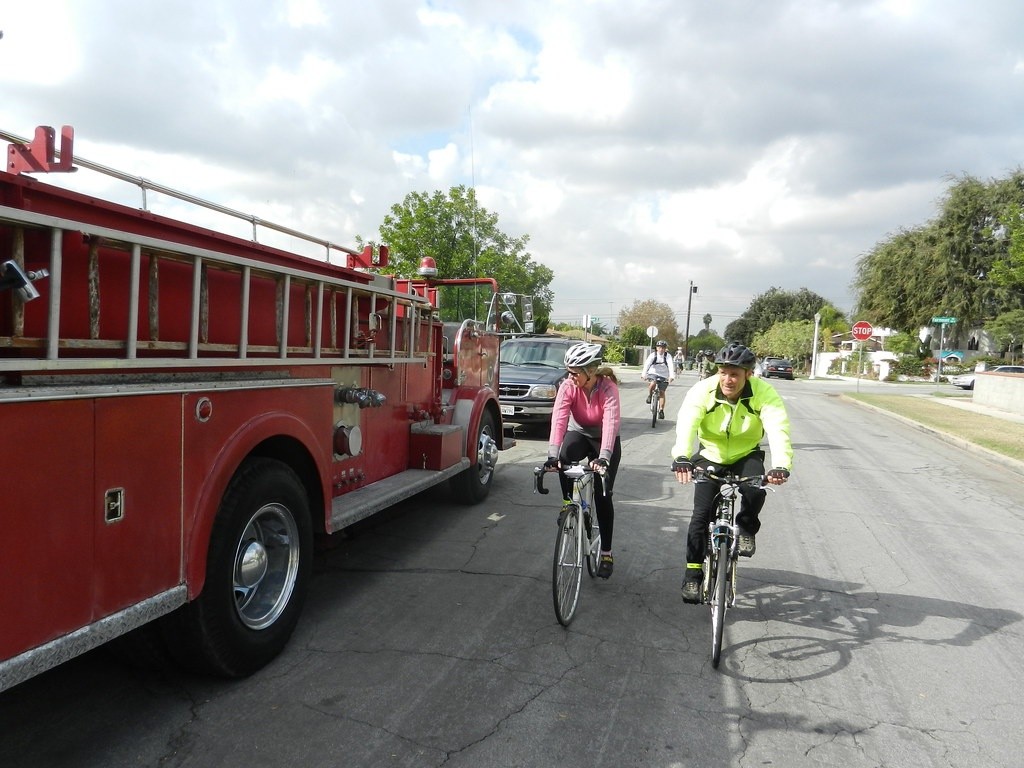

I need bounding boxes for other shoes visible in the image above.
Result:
[598,553,613,580]
[646,395,652,404]
[658,412,664,419]
[557,499,572,526]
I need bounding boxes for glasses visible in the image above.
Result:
[566,369,580,376]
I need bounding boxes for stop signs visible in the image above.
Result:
[852,320,873,342]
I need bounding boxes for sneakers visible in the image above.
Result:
[681,580,701,603]
[737,534,756,558]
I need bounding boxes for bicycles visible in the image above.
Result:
[670,463,778,670]
[696,361,712,381]
[530,462,610,628]
[674,361,684,379]
[640,375,670,428]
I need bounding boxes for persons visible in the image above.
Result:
[673,349,762,380]
[543,342,622,577]
[641,341,676,419]
[671,343,794,605]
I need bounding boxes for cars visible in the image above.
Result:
[764,360,794,380]
[951,365,1024,390]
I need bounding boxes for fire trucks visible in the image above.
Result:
[2,129,532,703]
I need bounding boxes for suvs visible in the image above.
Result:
[760,356,782,377]
[494,338,591,428]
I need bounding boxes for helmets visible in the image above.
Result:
[655,340,667,347]
[564,343,603,368]
[714,343,757,370]
[704,349,714,356]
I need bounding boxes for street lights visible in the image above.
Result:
[806,312,823,380]
